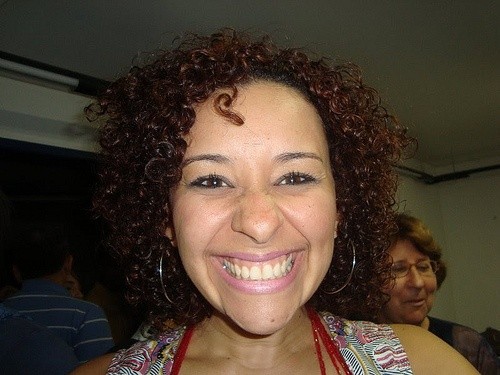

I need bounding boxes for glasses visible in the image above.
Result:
[388,258,440,278]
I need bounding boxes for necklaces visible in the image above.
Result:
[169,303,354,375]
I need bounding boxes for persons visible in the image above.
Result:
[382,214,500,375]
[0,227,136,375]
[68,23,481,375]
